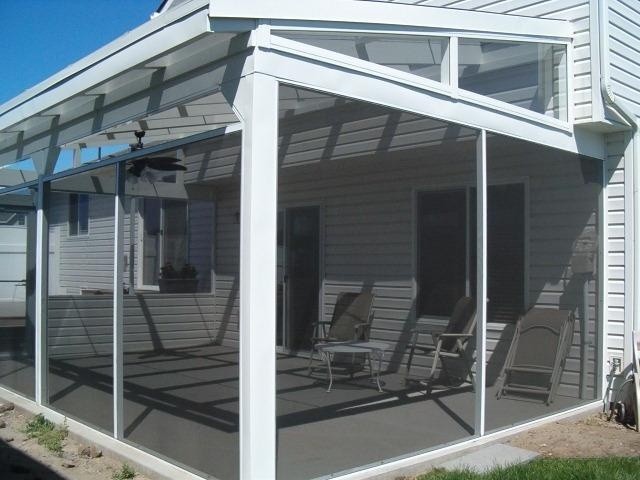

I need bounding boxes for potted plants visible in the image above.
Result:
[158,264,199,293]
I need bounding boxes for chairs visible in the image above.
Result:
[495,307,575,406]
[307,292,375,379]
[401,296,478,400]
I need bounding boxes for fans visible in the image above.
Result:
[84,130,187,177]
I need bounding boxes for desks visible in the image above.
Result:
[313,341,391,393]
